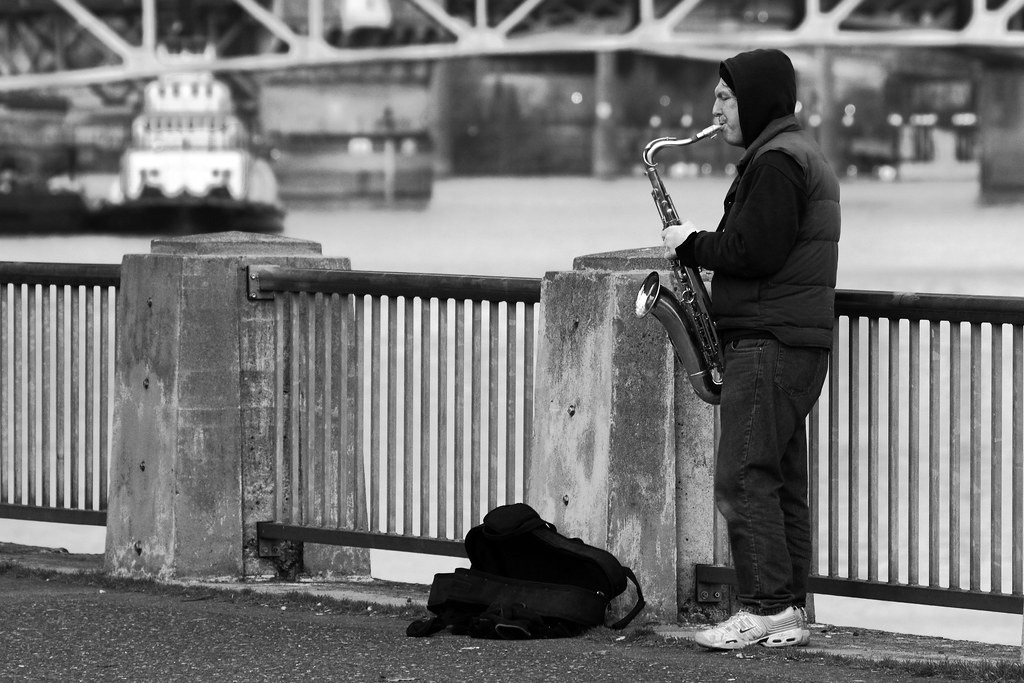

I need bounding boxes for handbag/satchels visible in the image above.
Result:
[405,502,645,642]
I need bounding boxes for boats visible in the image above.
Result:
[0,73,291,234]
[257,79,434,198]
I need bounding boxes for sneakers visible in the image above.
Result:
[694,607,810,650]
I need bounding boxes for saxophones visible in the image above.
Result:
[634,123,726,407]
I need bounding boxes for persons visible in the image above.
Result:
[661,49,842,649]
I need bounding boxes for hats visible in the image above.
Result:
[719,65,735,93]
